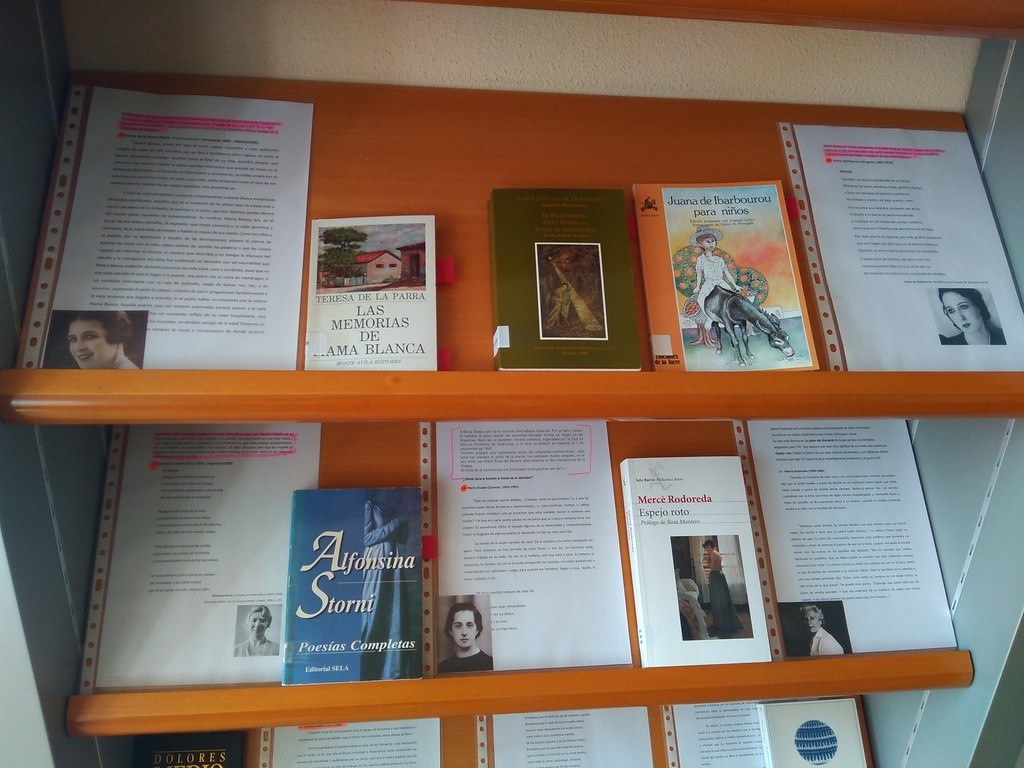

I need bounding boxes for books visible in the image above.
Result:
[304,215,438,371]
[632,181,819,372]
[756,695,874,768]
[281,486,423,687]
[487,187,641,371]
[132,731,244,768]
[618,456,772,668]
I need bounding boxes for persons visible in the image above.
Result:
[233,606,279,656]
[934,287,1005,345]
[703,540,743,632]
[437,602,492,672]
[359,488,423,681]
[799,603,844,656]
[63,311,140,370]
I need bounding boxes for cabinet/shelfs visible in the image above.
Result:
[0,1,1024,768]
